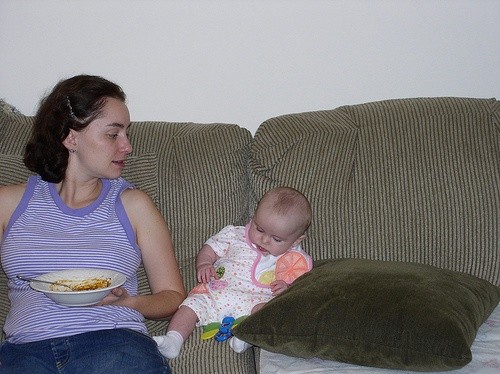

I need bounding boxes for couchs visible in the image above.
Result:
[1,97,499,374]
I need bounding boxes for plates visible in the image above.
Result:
[30,269,125,306]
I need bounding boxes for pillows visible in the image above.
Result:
[234,259,499,371]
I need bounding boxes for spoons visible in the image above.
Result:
[17,276,82,290]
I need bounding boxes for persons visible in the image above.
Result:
[0,75,187,374]
[153,187,313,359]
